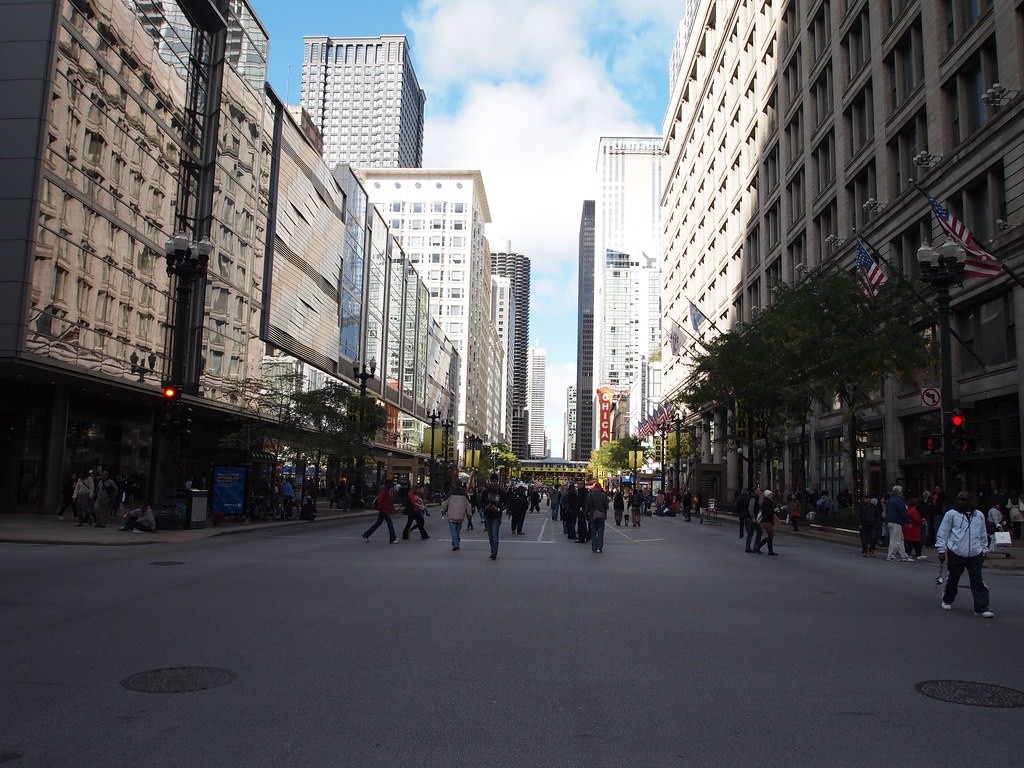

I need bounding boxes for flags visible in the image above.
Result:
[690,303,705,336]
[635,399,676,439]
[670,322,687,356]
[924,192,1002,278]
[855,241,888,297]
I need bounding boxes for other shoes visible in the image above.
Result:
[739,535,778,555]
[593,546,602,553]
[512,532,526,536]
[528,508,540,514]
[402,534,431,541]
[390,539,400,544]
[1005,553,1016,559]
[616,523,642,528]
[452,545,460,551]
[489,554,497,560]
[361,533,370,543]
[551,517,591,544]
[465,525,473,531]
[57,513,128,531]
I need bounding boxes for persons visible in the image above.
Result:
[319,476,368,508]
[57,471,156,531]
[261,475,317,520]
[782,488,853,531]
[935,491,994,617]
[549,481,610,553]
[361,479,400,544]
[185,470,209,490]
[396,481,431,540]
[440,473,544,560]
[855,482,1024,563]
[735,487,779,556]
[613,487,702,527]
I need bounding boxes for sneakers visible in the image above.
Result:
[941,600,952,610]
[973,610,994,618]
[862,548,927,562]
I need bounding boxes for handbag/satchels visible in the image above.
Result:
[592,508,606,521]
[793,511,802,518]
[373,496,378,505]
[988,531,1013,555]
[107,486,115,498]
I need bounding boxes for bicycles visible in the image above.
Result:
[249,496,299,521]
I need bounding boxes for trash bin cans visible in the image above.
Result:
[186,489,207,529]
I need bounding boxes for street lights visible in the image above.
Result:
[630,431,643,494]
[482,441,491,456]
[350,355,377,510]
[670,407,687,494]
[656,418,671,491]
[427,406,441,499]
[441,415,455,462]
[917,237,966,516]
[466,432,479,469]
[161,228,211,392]
[491,448,499,473]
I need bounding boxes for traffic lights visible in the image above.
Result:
[163,386,177,399]
[179,406,193,436]
[927,438,940,452]
[952,415,965,426]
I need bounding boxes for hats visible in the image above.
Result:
[862,493,871,497]
[764,490,772,496]
